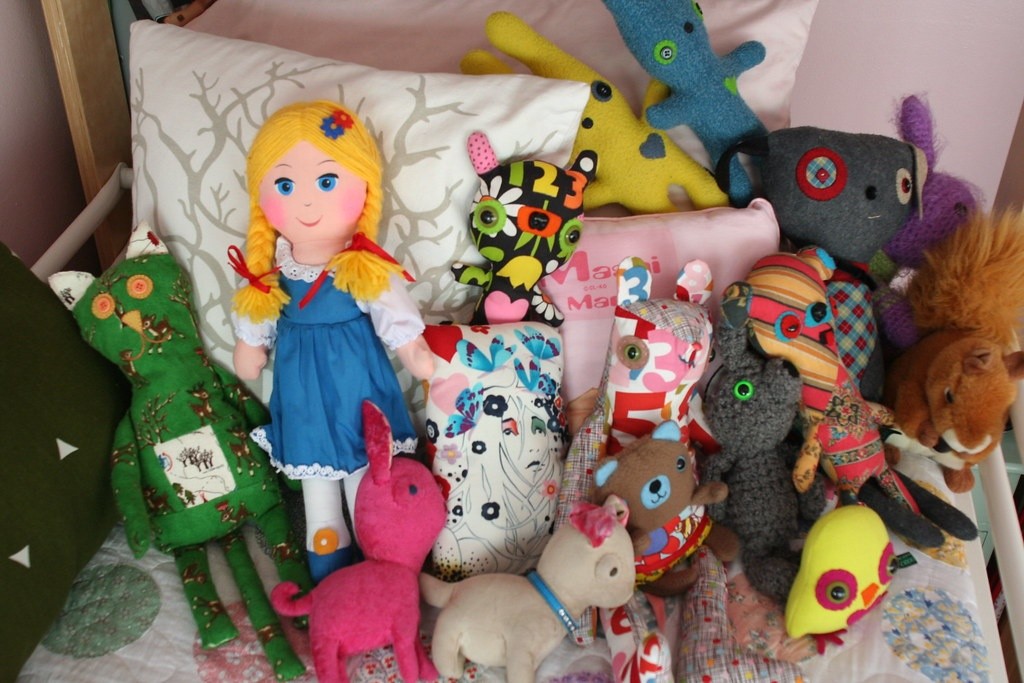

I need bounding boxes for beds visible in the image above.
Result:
[1,0,1024,682]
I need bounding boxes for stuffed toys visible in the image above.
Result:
[786,504,896,655]
[452,0,1024,683]
[270,399,451,683]
[48,219,311,683]
[420,494,636,683]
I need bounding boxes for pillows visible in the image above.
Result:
[124,18,593,407]
[0,241,132,681]
[540,197,780,433]
[408,322,572,579]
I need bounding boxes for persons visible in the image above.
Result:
[227,102,434,582]
